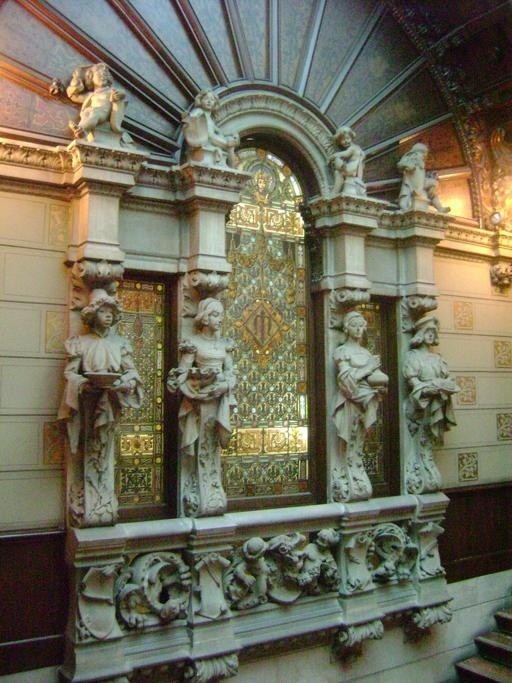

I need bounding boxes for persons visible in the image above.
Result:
[232,537,277,610]
[402,314,463,446]
[165,295,240,459]
[180,87,242,166]
[394,141,454,212]
[325,126,366,195]
[62,59,129,141]
[331,310,394,454]
[300,528,340,596]
[55,287,145,456]
